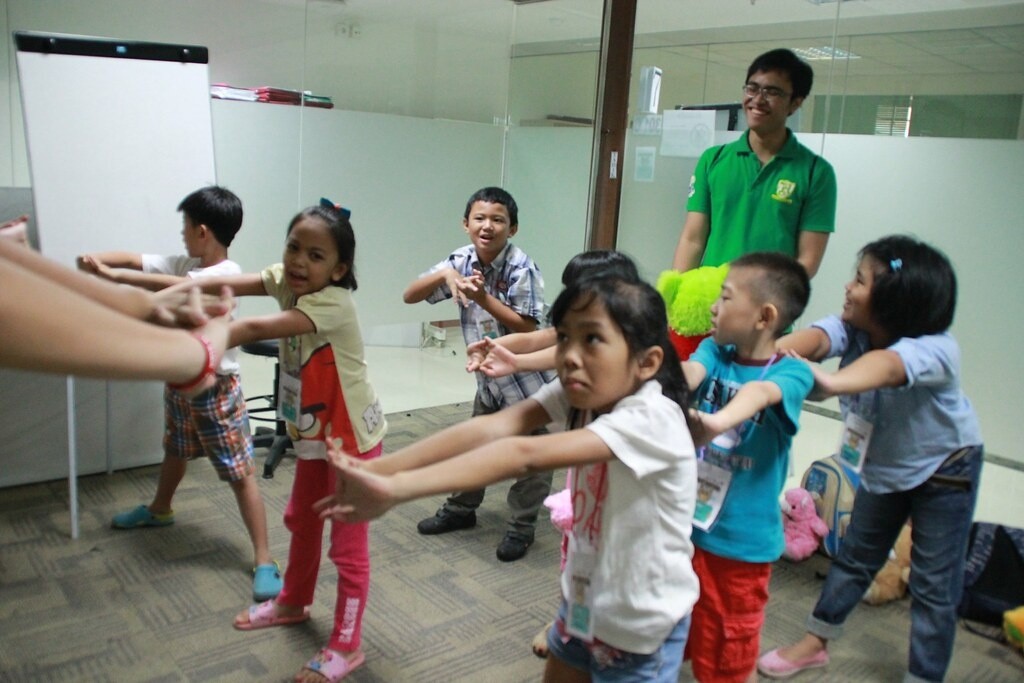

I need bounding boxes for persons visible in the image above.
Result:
[666,48,837,362]
[0,240,237,397]
[155,199,389,683]
[680,252,815,682]
[311,274,700,682]
[756,234,983,683]
[403,188,559,561]
[465,250,639,657]
[77,186,283,601]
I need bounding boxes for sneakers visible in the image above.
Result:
[416,506,478,536]
[497,534,538,562]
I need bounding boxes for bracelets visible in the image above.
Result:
[169,337,214,392]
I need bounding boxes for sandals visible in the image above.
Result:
[758,647,830,680]
[108,504,177,530]
[252,557,283,601]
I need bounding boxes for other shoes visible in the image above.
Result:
[743,83,795,102]
[531,619,563,659]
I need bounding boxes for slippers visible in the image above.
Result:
[296,646,367,683]
[232,600,312,631]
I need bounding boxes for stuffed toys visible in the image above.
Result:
[779,489,829,562]
[861,525,911,605]
[658,263,729,362]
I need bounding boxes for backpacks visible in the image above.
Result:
[800,454,861,559]
[956,521,1022,627]
